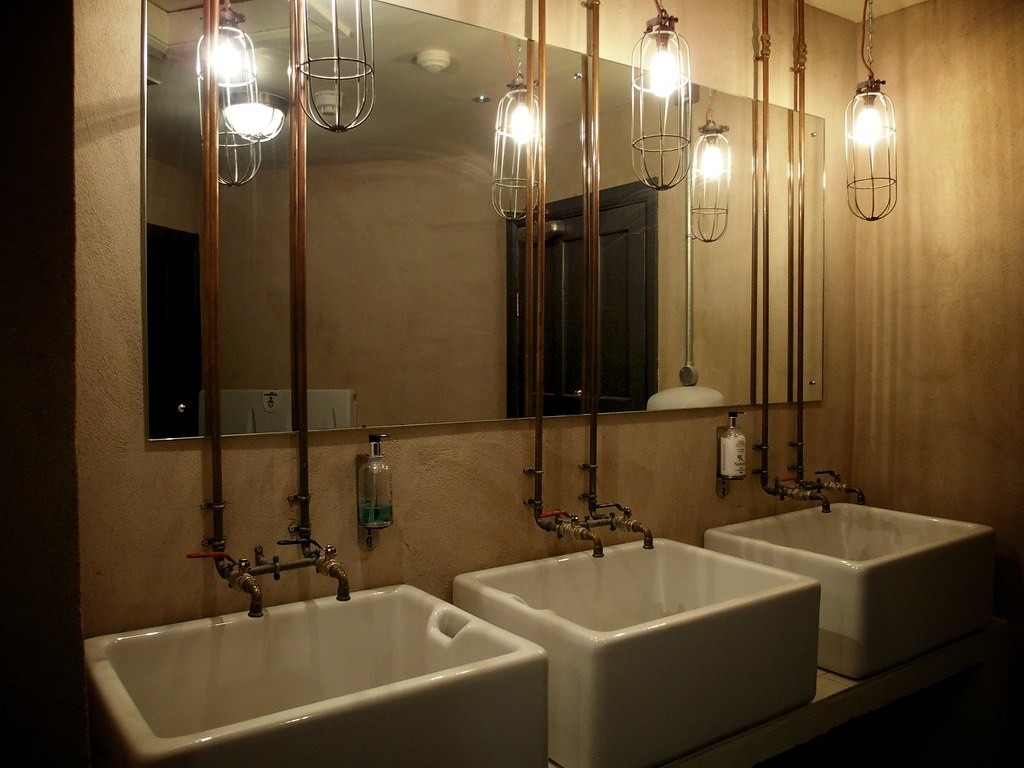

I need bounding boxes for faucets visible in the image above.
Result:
[537,509,604,558]
[814,469,866,505]
[597,502,655,549]
[185,550,264,618]
[780,476,832,513]
[276,538,351,602]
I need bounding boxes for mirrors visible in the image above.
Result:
[140,0,825,448]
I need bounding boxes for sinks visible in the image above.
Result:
[702,502,994,680]
[81,581,548,767]
[449,537,825,768]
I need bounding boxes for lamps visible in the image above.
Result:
[691,88,733,243]
[842,0,897,222]
[195,0,263,188]
[630,0,694,192]
[491,38,546,222]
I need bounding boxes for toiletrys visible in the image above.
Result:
[719,410,748,480]
[357,433,395,530]
